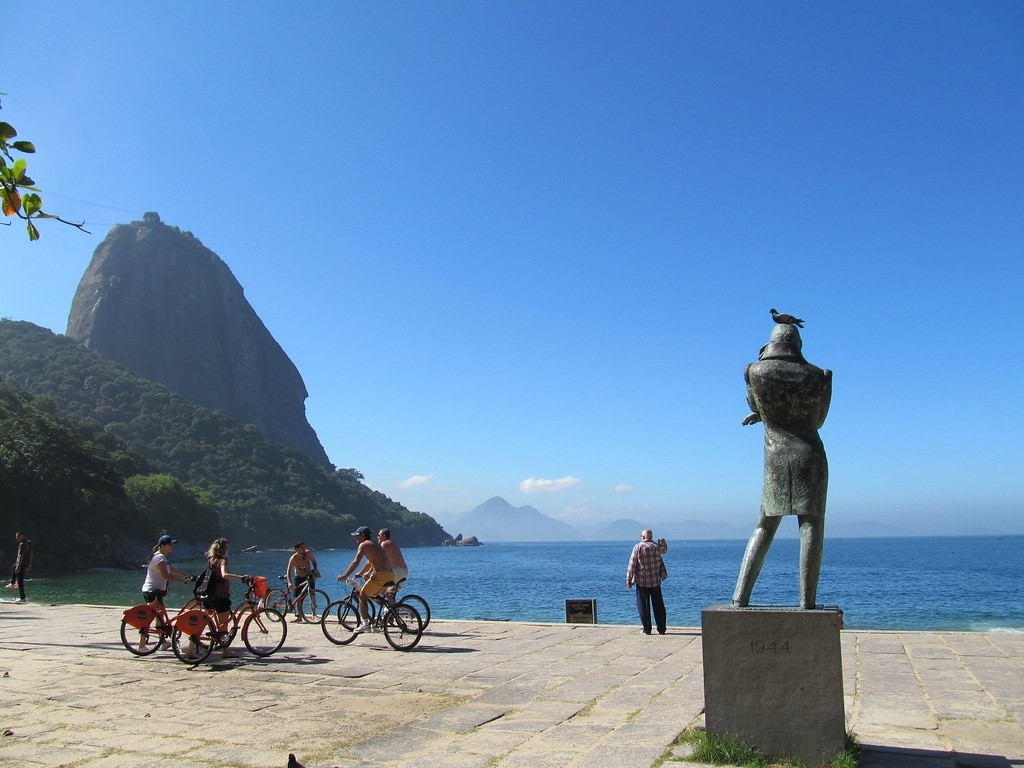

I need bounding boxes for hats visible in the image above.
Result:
[350,525,372,536]
[158,535,178,547]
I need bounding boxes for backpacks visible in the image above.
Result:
[193,557,227,601]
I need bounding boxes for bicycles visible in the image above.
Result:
[169,576,288,665]
[320,574,423,652]
[265,574,330,625]
[337,575,431,635]
[120,575,238,656]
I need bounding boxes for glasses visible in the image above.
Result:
[639,535,643,538]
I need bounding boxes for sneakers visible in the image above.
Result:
[384,607,390,615]
[354,623,371,634]
[138,646,155,653]
[161,641,173,651]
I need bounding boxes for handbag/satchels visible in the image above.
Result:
[161,584,169,596]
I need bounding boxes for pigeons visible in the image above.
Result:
[768,308,806,331]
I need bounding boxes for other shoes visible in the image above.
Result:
[291,618,302,623]
[312,615,321,620]
[222,652,239,658]
[185,647,199,659]
[17,598,26,602]
[640,629,648,635]
[5,583,13,589]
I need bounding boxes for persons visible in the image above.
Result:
[185,538,249,660]
[287,542,320,623]
[732,324,832,609]
[137,535,192,652]
[337,526,408,632]
[626,530,667,635]
[5,531,33,602]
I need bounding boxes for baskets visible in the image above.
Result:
[249,576,267,598]
